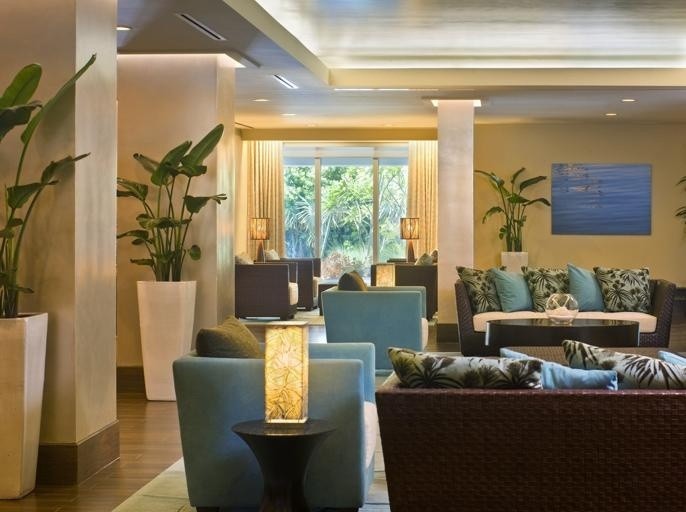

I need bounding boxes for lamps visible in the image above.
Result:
[400,217,420,262]
[263,320,310,424]
[250,218,269,262]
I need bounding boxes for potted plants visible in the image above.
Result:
[116,122,227,401]
[0,51,99,500]
[473,165,551,272]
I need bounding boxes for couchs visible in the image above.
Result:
[253,257,322,311]
[375,351,686,511]
[234,262,298,320]
[321,284,428,369]
[371,251,437,321]
[171,340,376,512]
[454,278,677,356]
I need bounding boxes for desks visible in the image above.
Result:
[230,419,337,512]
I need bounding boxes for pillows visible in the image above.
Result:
[658,349,686,366]
[387,346,545,390]
[500,348,619,391]
[562,339,686,389]
[455,262,653,314]
